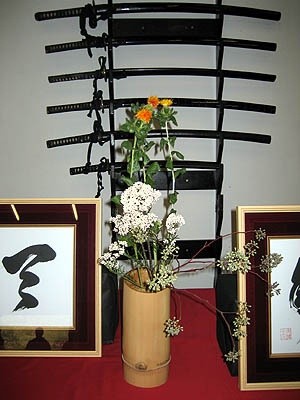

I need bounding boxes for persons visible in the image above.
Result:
[24,326,51,351]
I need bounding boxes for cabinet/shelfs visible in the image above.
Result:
[34,1,282,291]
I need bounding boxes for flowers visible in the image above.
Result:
[97,95,283,363]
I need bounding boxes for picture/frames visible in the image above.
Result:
[236,205,300,395]
[0,198,103,360]
[300,391,300,393]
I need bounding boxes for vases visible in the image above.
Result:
[121,268,171,389]
